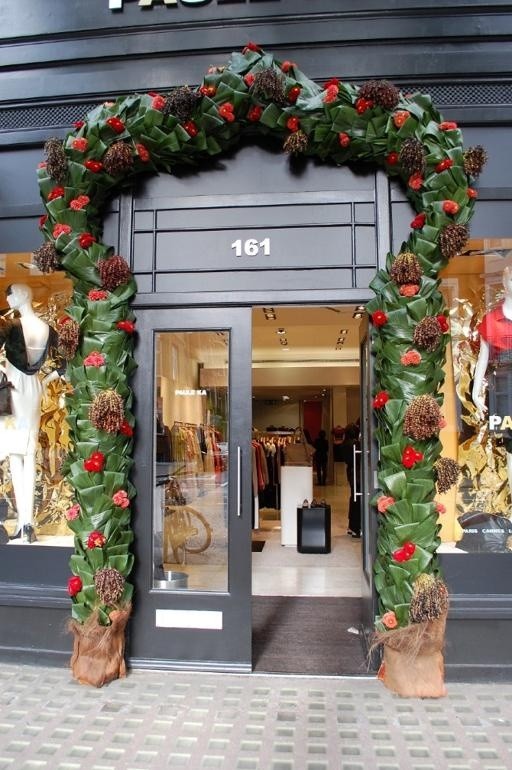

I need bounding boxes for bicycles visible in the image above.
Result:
[154,463,214,570]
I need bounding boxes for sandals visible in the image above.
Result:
[315,481,325,486]
[347,531,360,538]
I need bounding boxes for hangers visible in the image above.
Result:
[252,433,299,445]
[172,422,216,431]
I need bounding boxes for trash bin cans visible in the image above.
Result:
[154,571,188,589]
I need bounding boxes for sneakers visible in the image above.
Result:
[303,498,326,508]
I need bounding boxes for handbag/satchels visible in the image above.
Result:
[0,371,12,416]
[284,427,316,466]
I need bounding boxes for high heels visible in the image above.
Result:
[9,524,37,543]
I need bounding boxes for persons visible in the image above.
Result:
[315,430,329,485]
[472,264,512,502]
[341,422,361,538]
[0,283,67,544]
